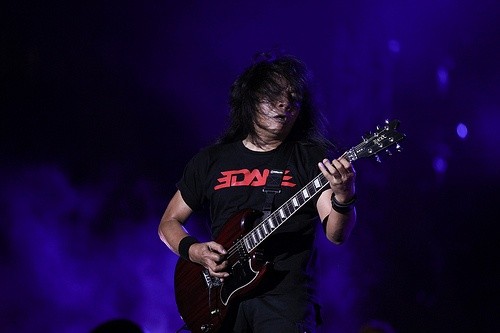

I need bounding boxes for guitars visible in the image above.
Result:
[174,119,407,333]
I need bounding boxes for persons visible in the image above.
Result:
[156,57,357,333]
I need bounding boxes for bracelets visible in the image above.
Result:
[331,192,357,207]
